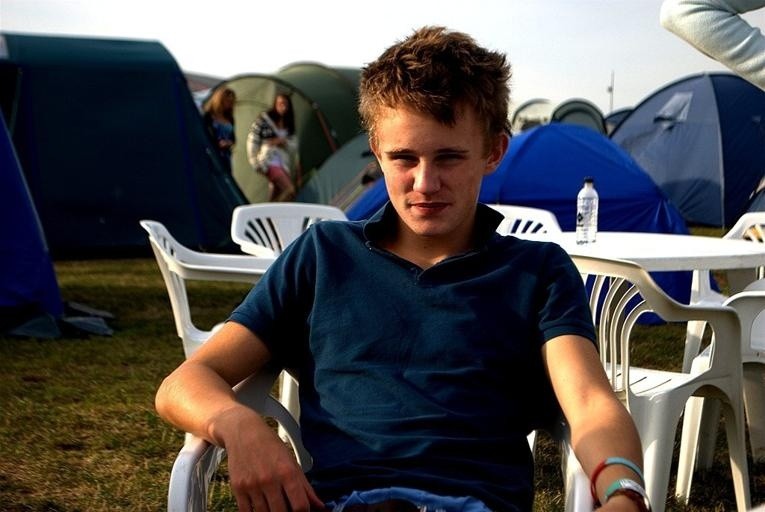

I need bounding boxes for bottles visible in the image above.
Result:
[576,176,598,247]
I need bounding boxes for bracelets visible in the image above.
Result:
[589,456,651,512]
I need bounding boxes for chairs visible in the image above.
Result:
[560,253,752,512]
[166,349,595,512]
[486,203,564,235]
[673,275,765,507]
[137,218,313,461]
[231,203,350,262]
[680,210,765,374]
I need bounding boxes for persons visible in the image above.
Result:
[154,25,652,512]
[297,75,764,326]
[659,0,765,93]
[250,94,296,204]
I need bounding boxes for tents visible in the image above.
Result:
[1,30,249,338]
[201,62,368,202]
